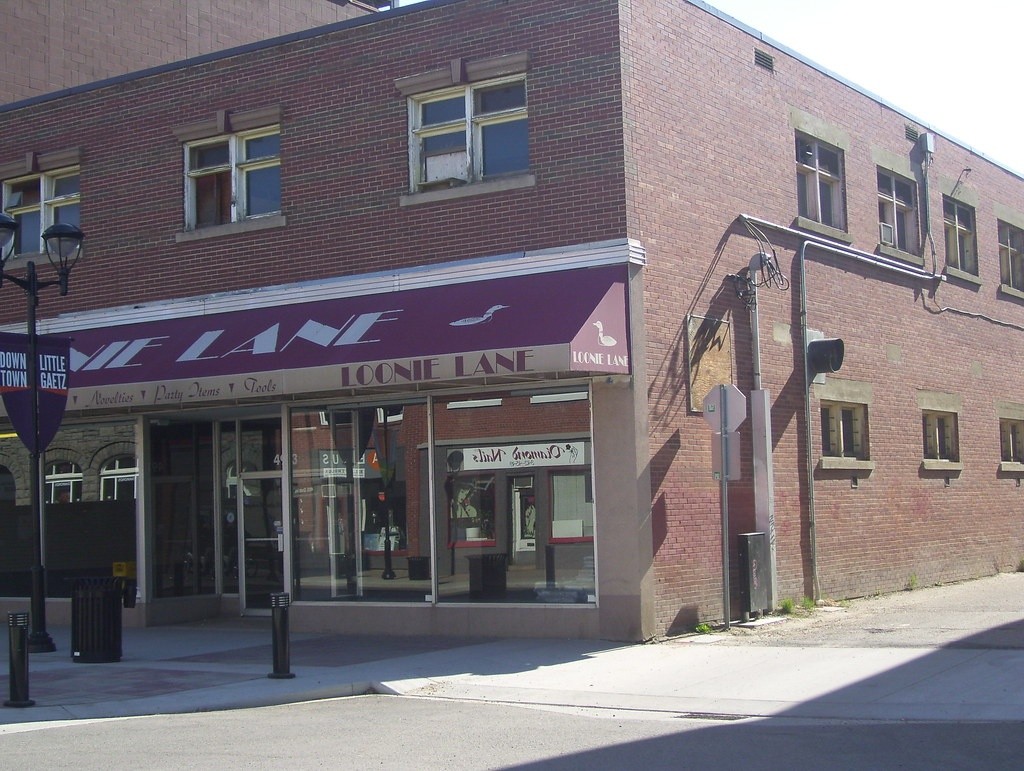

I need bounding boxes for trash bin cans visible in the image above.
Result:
[464,553,508,600]
[407,556,431,579]
[66,575,137,664]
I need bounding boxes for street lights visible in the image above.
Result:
[0,211,83,653]
